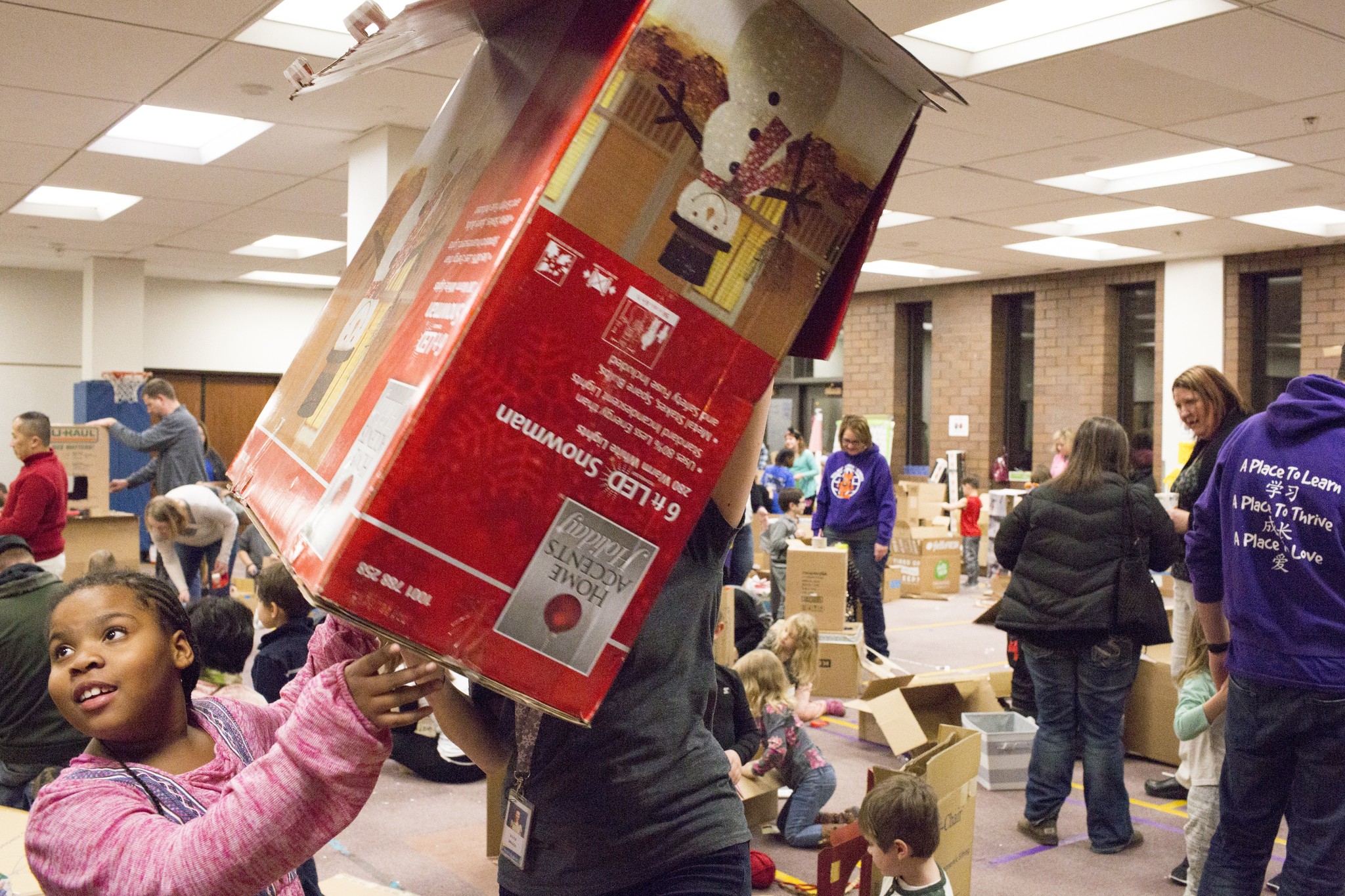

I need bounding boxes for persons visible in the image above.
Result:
[1003,631,1038,713]
[400,362,777,896]
[0,378,314,810]
[710,425,863,849]
[994,418,1184,853]
[1184,342,1345,896]
[25,564,443,896]
[856,774,953,896]
[1144,366,1283,896]
[1129,428,1157,493]
[811,414,897,659]
[993,446,1011,490]
[1024,426,1075,494]
[510,809,522,836]
[941,477,982,590]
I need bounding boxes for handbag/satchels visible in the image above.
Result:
[1114,484,1174,647]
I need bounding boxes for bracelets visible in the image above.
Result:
[1208,641,1229,653]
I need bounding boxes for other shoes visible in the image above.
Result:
[959,580,978,588]
[1266,872,1284,892]
[1144,776,1190,800]
[1090,829,1143,854]
[1017,818,1060,846]
[826,698,846,716]
[1170,855,1189,883]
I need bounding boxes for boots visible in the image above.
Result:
[823,805,860,824]
[820,823,849,849]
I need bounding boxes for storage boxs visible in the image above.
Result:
[34,0,1193,896]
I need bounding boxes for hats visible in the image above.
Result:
[0,534,34,557]
